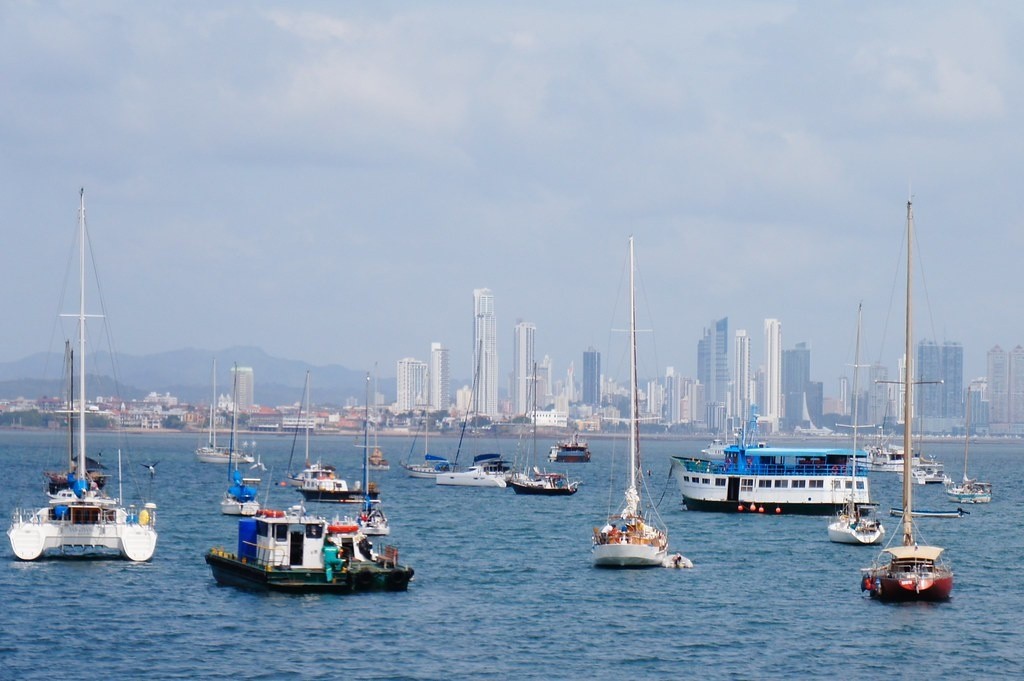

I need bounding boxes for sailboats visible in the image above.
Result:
[192,354,255,466]
[591,233,670,566]
[215,361,263,516]
[471,351,513,473]
[361,383,391,470]
[399,370,461,479]
[507,362,582,496]
[281,362,382,503]
[944,383,993,505]
[854,382,920,471]
[434,338,517,488]
[898,373,949,485]
[826,304,886,544]
[862,198,954,601]
[5,186,159,563]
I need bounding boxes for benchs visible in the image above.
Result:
[375,544,399,570]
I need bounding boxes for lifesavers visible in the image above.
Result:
[257,509,285,517]
[139,510,149,524]
[832,466,838,474]
[726,459,731,467]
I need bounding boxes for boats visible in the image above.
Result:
[548,431,590,464]
[203,502,413,597]
[668,405,876,517]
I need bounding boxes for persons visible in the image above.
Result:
[322,541,345,582]
[607,524,617,544]
[342,539,354,570]
[359,537,373,560]
[856,519,881,533]
